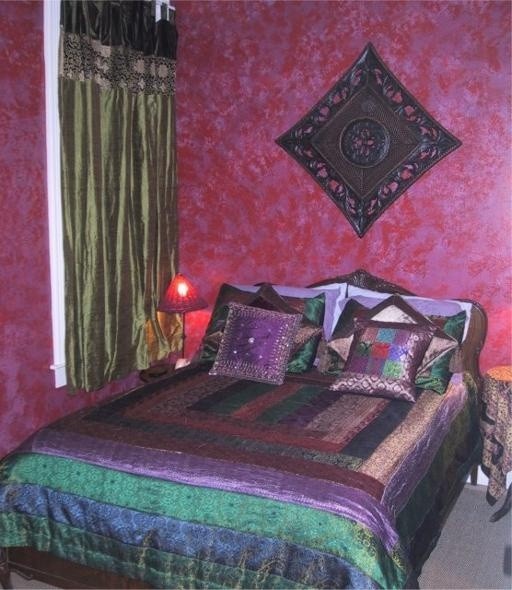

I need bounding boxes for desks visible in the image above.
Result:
[485,364,511,577]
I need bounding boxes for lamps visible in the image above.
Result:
[155,272,209,373]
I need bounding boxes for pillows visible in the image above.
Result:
[190,281,474,404]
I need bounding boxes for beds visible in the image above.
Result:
[0,270,489,590]
